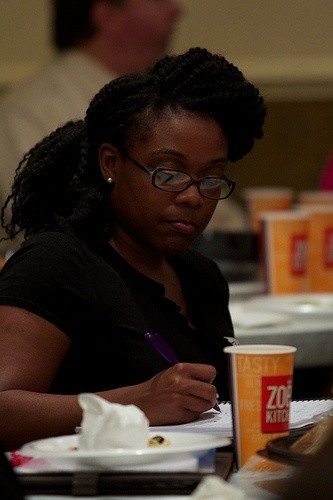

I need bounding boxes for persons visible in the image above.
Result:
[1,45,267,448]
[0,0,252,256]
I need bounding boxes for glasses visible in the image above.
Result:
[118,150,236,201]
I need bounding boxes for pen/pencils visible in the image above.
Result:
[145,332,224,414]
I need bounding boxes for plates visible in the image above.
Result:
[20,430,230,470]
[265,294,333,316]
[229,308,289,328]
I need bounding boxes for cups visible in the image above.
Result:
[297,192,333,205]
[256,210,314,294]
[223,344,297,472]
[295,204,333,294]
[244,187,293,232]
